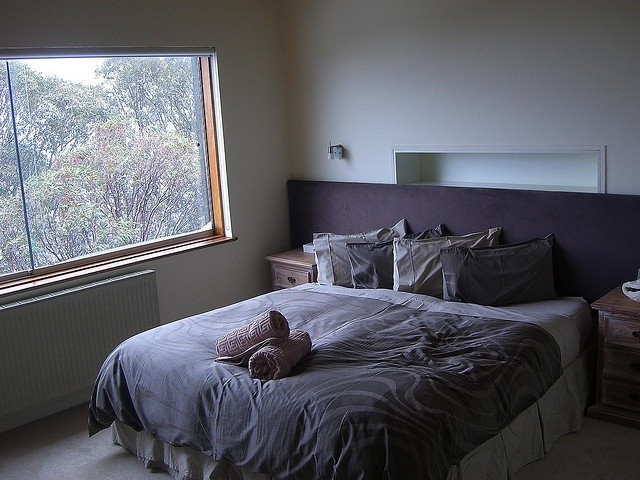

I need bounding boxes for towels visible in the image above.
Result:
[213,310,290,367]
[248,328,312,380]
[622,278,640,304]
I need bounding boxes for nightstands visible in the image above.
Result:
[586,286,640,430]
[264,245,318,291]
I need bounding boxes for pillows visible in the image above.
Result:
[312,217,413,288]
[440,232,559,306]
[345,223,446,289]
[392,226,502,299]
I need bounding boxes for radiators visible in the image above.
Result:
[0,268,160,433]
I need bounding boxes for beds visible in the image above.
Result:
[87,182,640,480]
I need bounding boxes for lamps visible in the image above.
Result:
[327,140,338,160]
